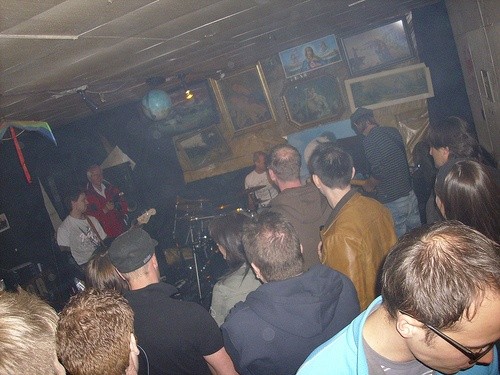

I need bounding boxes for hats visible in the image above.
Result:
[351,106,371,123]
[108,228,159,274]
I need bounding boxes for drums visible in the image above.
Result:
[188,216,214,246]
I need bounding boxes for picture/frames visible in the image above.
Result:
[158,15,434,171]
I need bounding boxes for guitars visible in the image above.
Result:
[133,208,156,228]
[306,177,374,194]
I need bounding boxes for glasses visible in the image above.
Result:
[398,310,500,364]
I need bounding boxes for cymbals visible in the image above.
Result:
[233,185,266,200]
[190,198,215,203]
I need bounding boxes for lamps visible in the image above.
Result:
[78,92,100,112]
[182,81,193,100]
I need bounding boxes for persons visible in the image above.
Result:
[109,228,238,375]
[0,106,500,375]
[293,218,500,375]
[221,212,362,375]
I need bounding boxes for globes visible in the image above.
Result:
[141,76,173,121]
